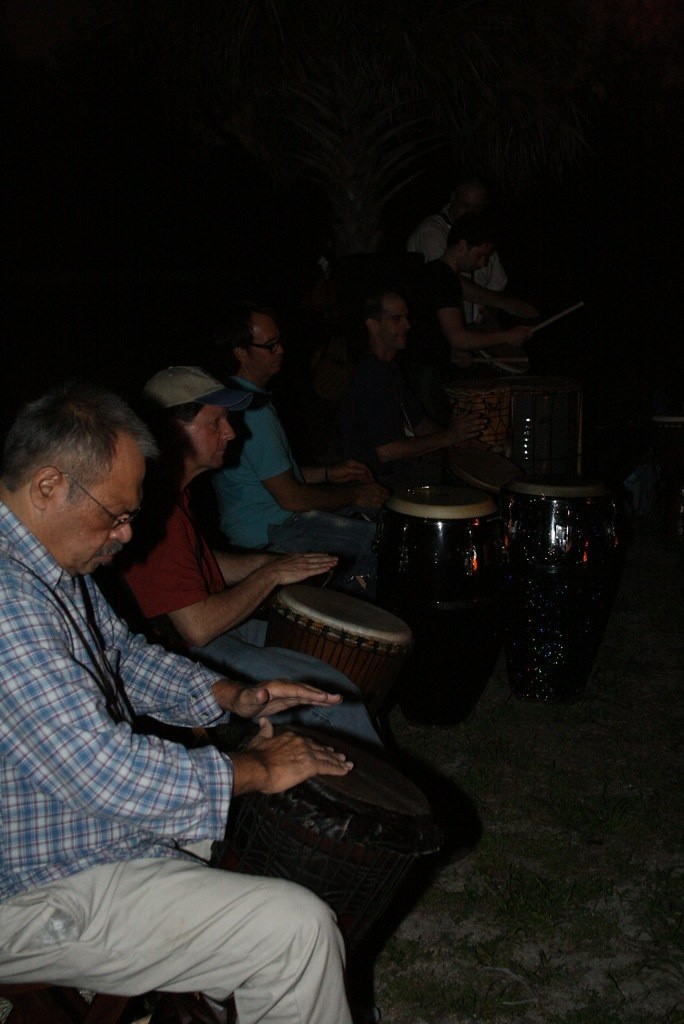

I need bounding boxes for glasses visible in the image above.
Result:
[245,335,279,355]
[55,469,141,532]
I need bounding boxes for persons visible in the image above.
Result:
[99,364,386,759]
[213,311,424,626]
[0,384,355,1024]
[346,180,542,484]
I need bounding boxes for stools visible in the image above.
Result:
[0,982,132,1024]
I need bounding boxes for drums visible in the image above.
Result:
[263,584,412,723]
[379,487,509,727]
[441,378,511,447]
[504,473,635,705]
[441,442,523,499]
[147,728,445,1024]
[652,415,684,552]
[479,366,583,482]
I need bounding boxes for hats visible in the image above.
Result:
[143,365,253,414]
